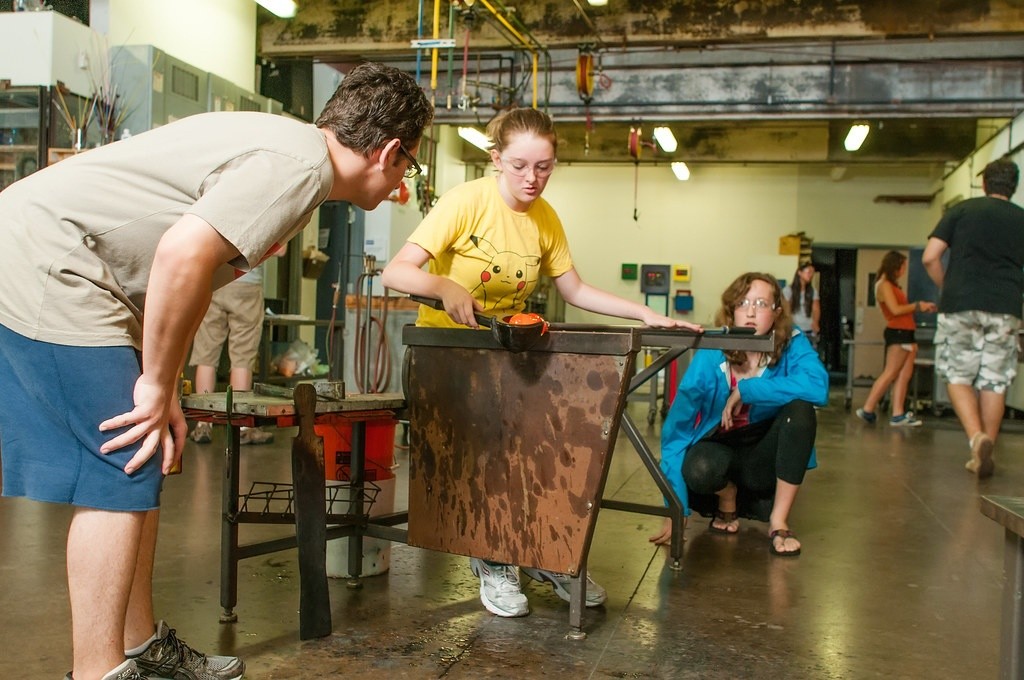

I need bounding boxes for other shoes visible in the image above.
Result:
[966,433,996,481]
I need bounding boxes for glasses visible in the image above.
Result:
[734,297,778,314]
[397,142,422,178]
[498,154,558,177]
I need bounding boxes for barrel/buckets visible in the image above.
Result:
[319,410,397,578]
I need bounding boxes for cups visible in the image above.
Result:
[101,130,115,146]
[71,128,86,149]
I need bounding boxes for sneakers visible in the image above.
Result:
[469,555,529,617]
[125,619,246,680]
[889,411,921,427]
[856,409,877,426]
[239,426,273,446]
[520,564,607,607]
[64,659,174,680]
[190,424,213,444]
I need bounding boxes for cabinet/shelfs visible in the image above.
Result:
[216,315,345,389]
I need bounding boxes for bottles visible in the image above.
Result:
[121,128,132,140]
[12,0,40,11]
[8,129,18,145]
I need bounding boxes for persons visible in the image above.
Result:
[921,159,1024,480]
[0,60,433,680]
[856,250,936,427]
[647,270,828,556]
[381,108,701,618]
[779,262,820,338]
[189,241,287,445]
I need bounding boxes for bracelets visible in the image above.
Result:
[915,301,920,312]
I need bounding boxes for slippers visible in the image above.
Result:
[708,510,740,534]
[769,529,802,556]
